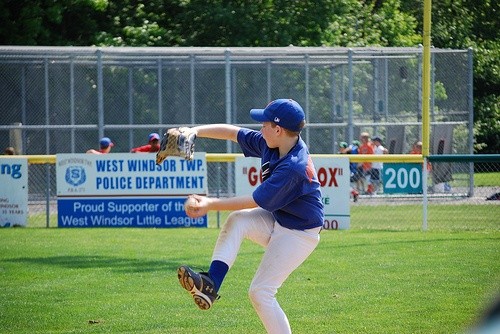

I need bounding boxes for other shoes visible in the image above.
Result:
[354,192,359,202]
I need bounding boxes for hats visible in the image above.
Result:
[100,138,113,147]
[372,136,383,143]
[340,141,348,149]
[352,141,360,148]
[149,133,159,141]
[250,98,305,131]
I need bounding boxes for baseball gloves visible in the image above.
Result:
[156,126,197,166]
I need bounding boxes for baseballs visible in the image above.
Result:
[184,197,199,216]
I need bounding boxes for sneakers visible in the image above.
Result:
[178,264,221,310]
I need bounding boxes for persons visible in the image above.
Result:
[4,148,15,155]
[158,98,327,333]
[408,141,432,175]
[336,130,390,203]
[130,132,161,154]
[87,137,114,153]
